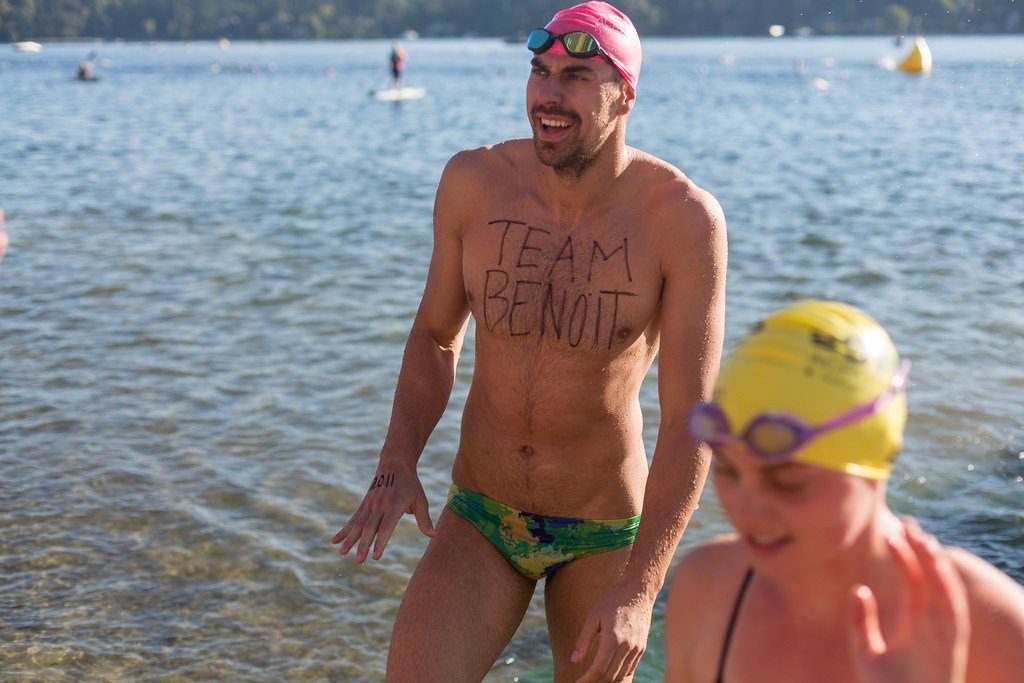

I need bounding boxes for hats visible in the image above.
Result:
[711,298,908,479]
[542,1,642,94]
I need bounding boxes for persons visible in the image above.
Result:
[663,299,1024,683]
[389,41,406,87]
[330,1,728,683]
[76,50,99,82]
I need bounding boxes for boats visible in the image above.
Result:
[373,88,426,105]
[892,35,931,75]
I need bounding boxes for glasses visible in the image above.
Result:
[689,360,915,464]
[527,28,615,67]
